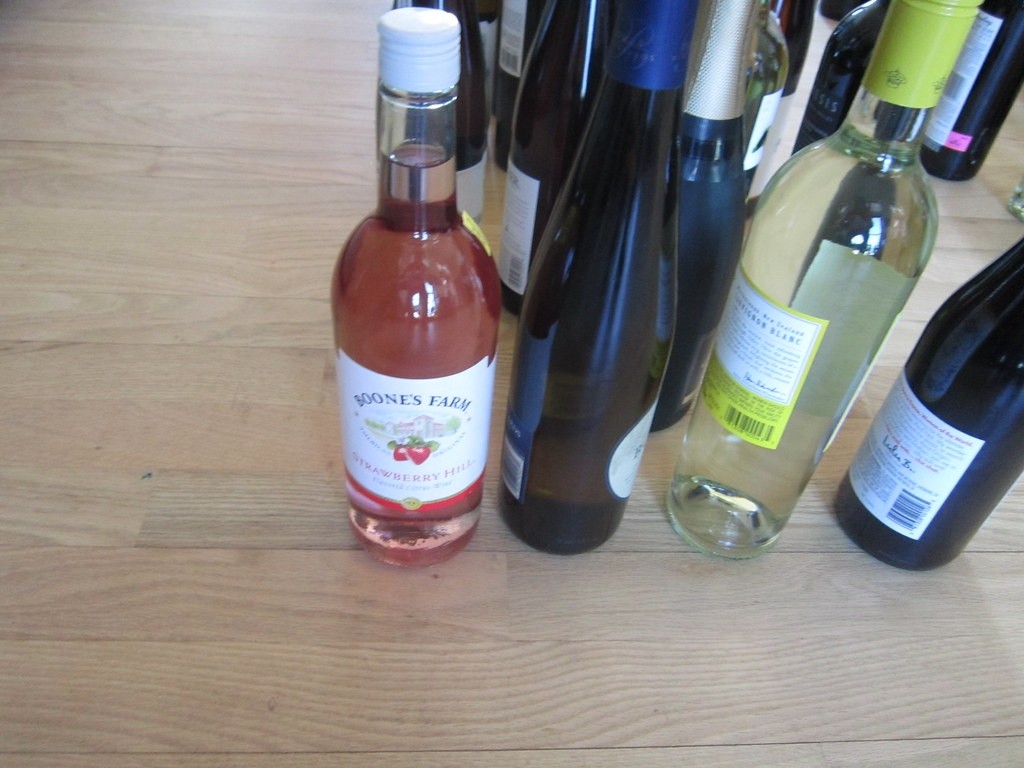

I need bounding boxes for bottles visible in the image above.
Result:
[333,1,1024,570]
